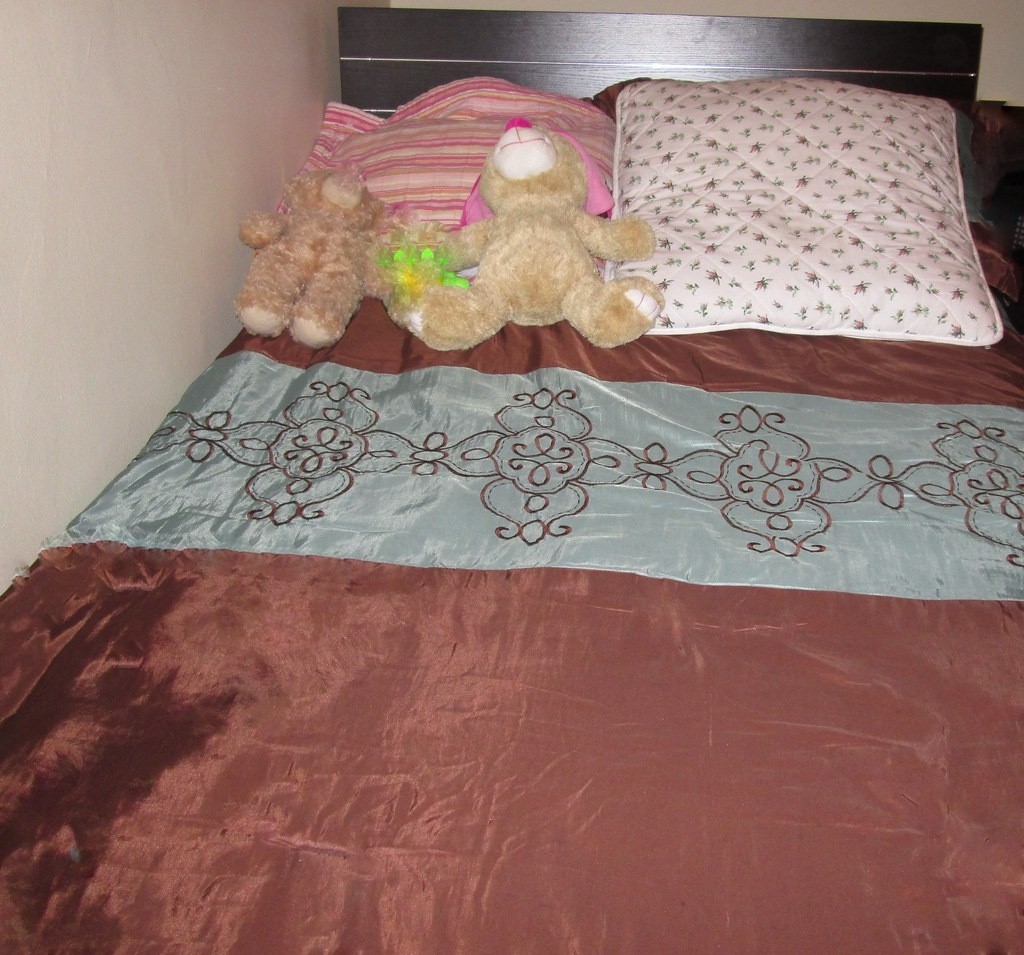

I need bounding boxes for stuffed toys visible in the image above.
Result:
[237,167,386,345]
[382,116,664,353]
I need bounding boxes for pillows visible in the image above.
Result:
[606,75,1005,348]
[276,76,616,227]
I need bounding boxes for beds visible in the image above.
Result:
[0,6,1024,955]
